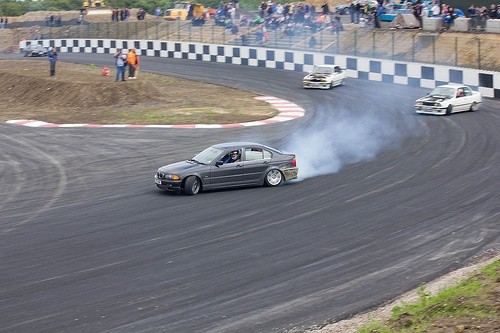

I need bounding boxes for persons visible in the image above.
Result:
[111,7,129,23]
[412,0,423,18]
[49,12,62,27]
[126,48,137,79]
[230,1,344,49]
[113,49,128,82]
[440,2,500,27]
[349,1,362,24]
[48,48,57,76]
[230,151,240,163]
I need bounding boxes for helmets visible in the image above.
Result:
[230,150,240,160]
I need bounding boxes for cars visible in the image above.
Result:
[22,44,49,57]
[302,64,348,90]
[334,0,380,15]
[414,84,482,116]
[154,141,299,196]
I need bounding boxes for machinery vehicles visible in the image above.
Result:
[162,1,211,24]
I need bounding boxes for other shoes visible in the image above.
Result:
[129,76,136,79]
